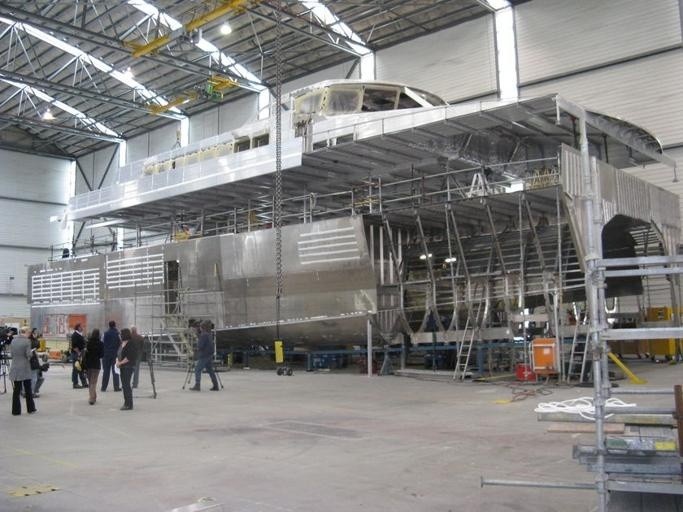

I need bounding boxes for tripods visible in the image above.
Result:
[182,351,225,389]
[0,341,14,393]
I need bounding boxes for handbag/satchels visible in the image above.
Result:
[114,359,120,374]
[29,351,39,370]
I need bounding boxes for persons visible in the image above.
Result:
[71,321,144,410]
[6,326,49,416]
[189,322,219,390]
